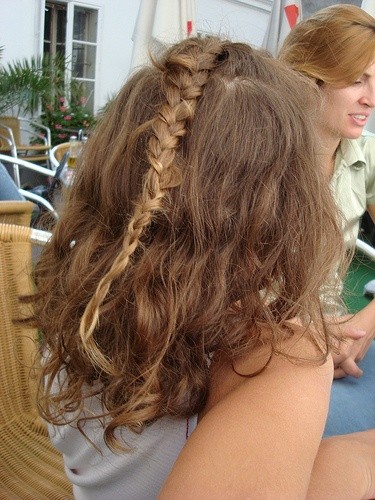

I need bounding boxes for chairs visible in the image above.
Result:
[0,114,86,500]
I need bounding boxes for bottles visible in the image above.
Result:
[80,136,88,152]
[68,136,79,170]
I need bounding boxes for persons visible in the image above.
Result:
[31,32,375,500]
[275,5,375,381]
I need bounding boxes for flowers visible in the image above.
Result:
[31,86,97,154]
